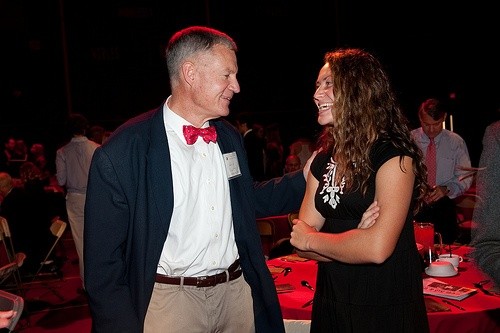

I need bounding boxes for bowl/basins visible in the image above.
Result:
[424,261,458,277]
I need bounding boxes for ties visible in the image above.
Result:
[426,138,436,186]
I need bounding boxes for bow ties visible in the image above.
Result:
[183,125,217,145]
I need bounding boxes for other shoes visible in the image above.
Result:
[78,289,86,295]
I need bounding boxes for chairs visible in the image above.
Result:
[0,215,67,333]
[255,211,301,259]
[452,192,485,239]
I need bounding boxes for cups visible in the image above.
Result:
[413,222,434,259]
[436,254,463,268]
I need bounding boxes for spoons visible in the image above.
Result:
[284,267,291,276]
[473,282,490,294]
[301,281,314,290]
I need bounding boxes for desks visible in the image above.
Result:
[259,240,500,333]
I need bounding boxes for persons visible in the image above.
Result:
[285,154,299,174]
[84,24,323,333]
[234,116,284,182]
[403,97,474,246]
[288,48,430,333]
[470,121,500,285]
[0,112,112,333]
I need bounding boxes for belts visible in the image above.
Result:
[155,259,243,288]
